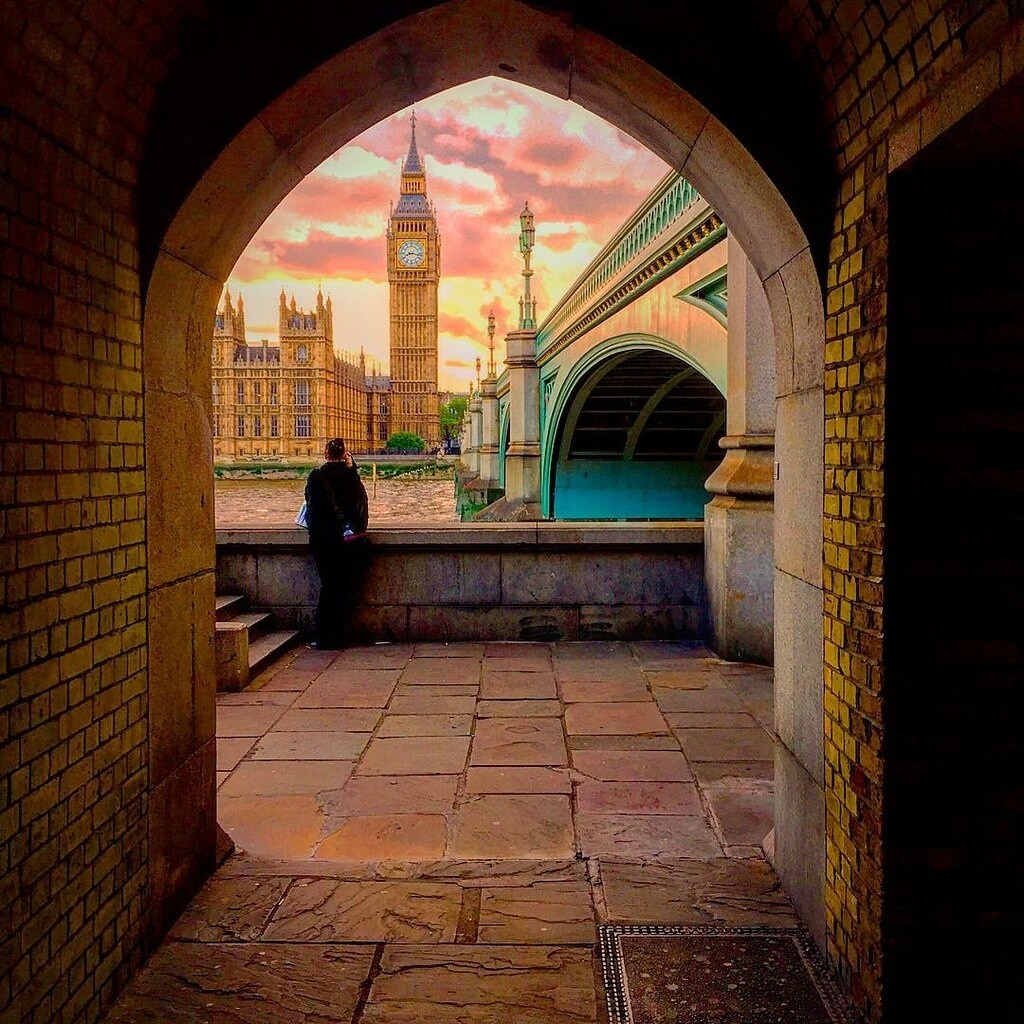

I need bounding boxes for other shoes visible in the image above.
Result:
[315,640,350,650]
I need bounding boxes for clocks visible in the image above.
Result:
[397,239,425,267]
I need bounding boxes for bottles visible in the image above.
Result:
[344,525,354,536]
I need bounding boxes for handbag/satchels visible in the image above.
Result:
[340,533,373,574]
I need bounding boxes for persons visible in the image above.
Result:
[437,449,442,455]
[401,449,406,455]
[304,438,369,650]
[439,440,444,457]
